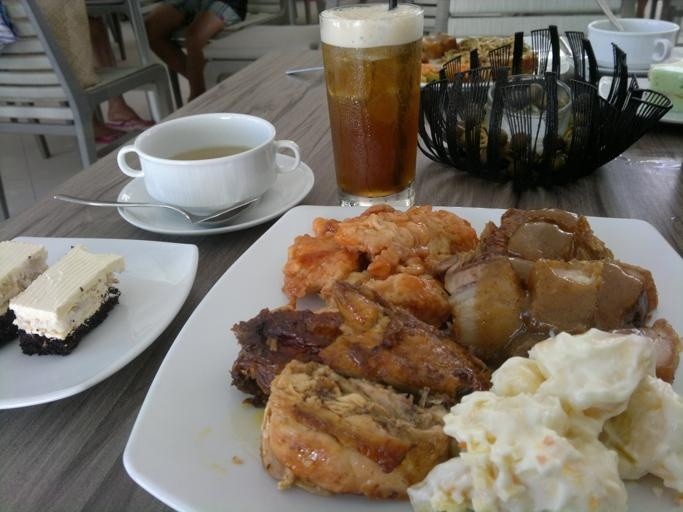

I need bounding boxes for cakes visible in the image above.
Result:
[1,242,48,345]
[11,247,124,356]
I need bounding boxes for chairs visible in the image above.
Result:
[434,0,637,38]
[391,0,454,34]
[169,0,289,110]
[0,0,173,223]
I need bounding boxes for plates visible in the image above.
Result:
[0,237,201,411]
[422,34,570,88]
[121,203,683,508]
[594,67,673,76]
[598,76,683,126]
[117,154,314,237]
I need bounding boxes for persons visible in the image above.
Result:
[86,11,157,143]
[145,1,248,105]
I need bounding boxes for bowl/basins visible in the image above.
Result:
[487,74,573,158]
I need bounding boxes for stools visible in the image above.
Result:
[201,23,321,97]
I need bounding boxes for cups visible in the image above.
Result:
[586,18,678,68]
[318,4,425,214]
[114,113,301,220]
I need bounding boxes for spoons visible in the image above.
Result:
[50,191,260,227]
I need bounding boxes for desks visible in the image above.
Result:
[86,0,182,123]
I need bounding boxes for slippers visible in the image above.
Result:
[96,131,126,142]
[109,119,156,131]
